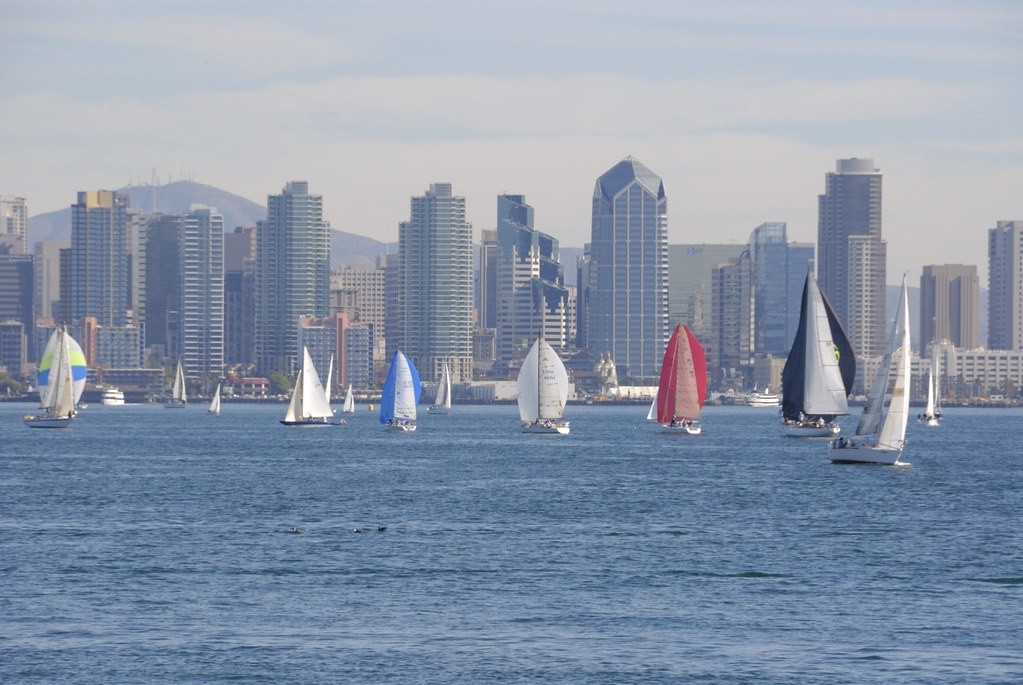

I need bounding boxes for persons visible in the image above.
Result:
[816,417,825,427]
[670,415,678,428]
[798,411,804,423]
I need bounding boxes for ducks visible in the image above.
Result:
[291,527,305,533]
[352,528,361,533]
[377,526,388,531]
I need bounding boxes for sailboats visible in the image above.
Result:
[514,331,572,434]
[916,355,943,426]
[279,344,355,428]
[164,359,187,408]
[206,383,222,415]
[379,349,422,431]
[780,265,858,436]
[425,361,452,414]
[646,321,709,435]
[823,272,913,466]
[22,321,89,429]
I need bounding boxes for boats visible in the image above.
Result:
[100,388,125,407]
